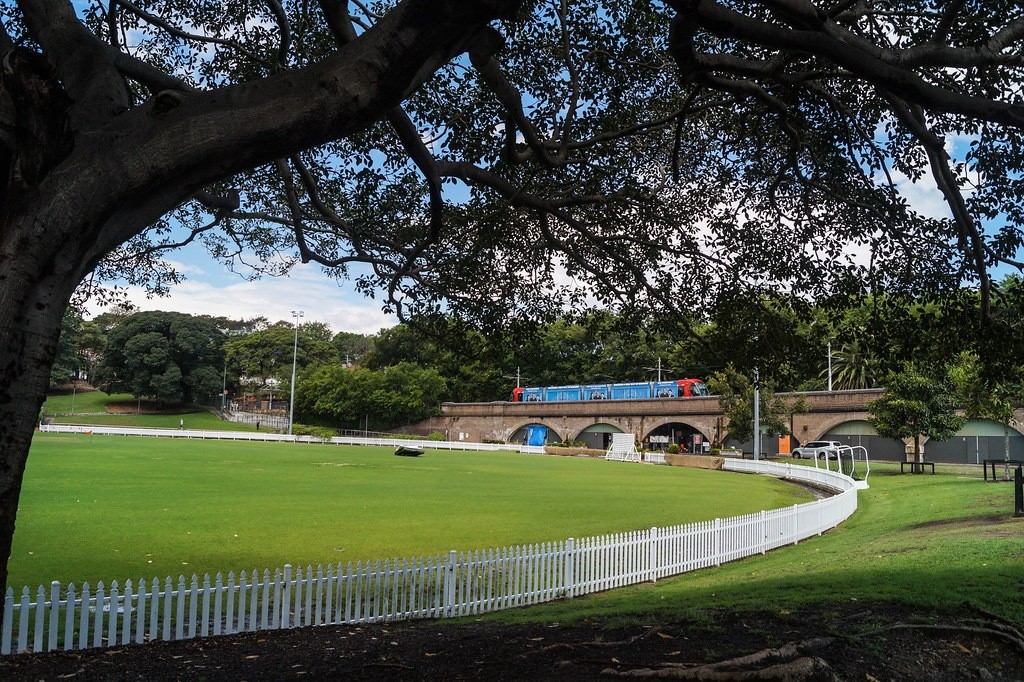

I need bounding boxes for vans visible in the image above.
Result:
[792,441,844,460]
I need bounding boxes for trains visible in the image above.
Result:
[509,379,710,402]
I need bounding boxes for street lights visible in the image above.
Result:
[288,310,304,435]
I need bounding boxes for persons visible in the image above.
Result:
[668,389,674,397]
[531,393,536,401]
[526,395,530,401]
[589,393,594,400]
[594,392,601,399]
[256,422,260,430]
[660,387,669,397]
[180,418,183,430]
[601,393,606,399]
[656,390,661,398]
[536,395,541,401]
